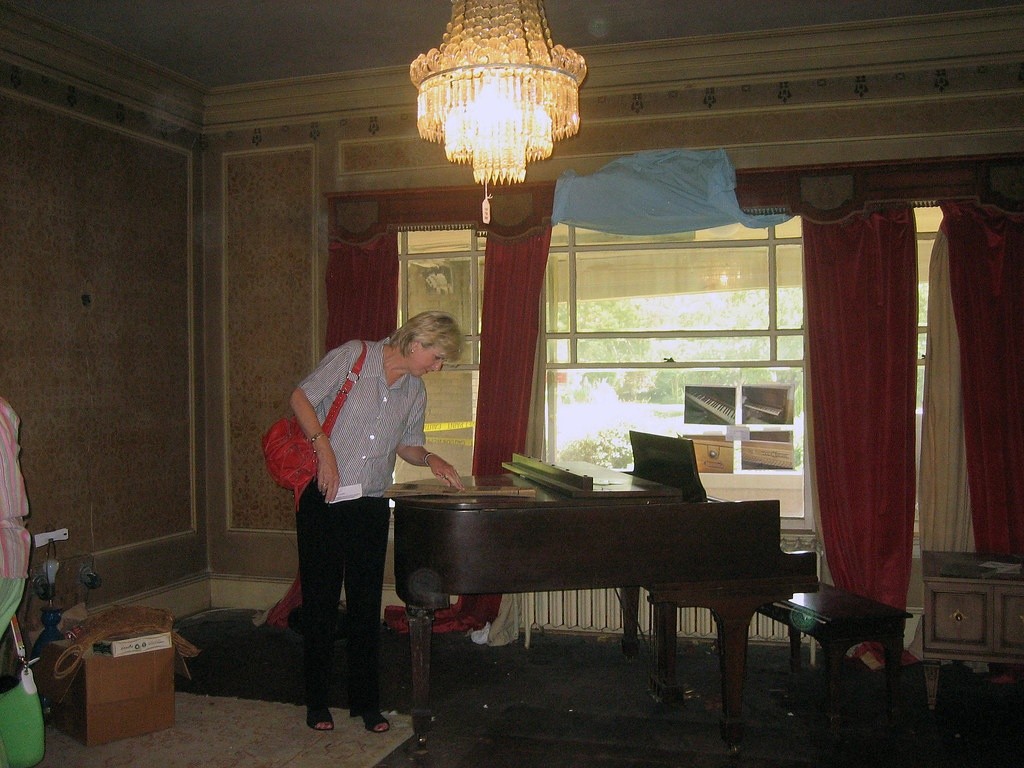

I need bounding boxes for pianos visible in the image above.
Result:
[743,387,789,424]
[685,386,735,425]
[389,428,824,768]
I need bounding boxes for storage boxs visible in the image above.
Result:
[40,638,176,748]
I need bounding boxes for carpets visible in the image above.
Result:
[31,692,417,768]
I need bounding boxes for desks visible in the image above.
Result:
[922,550,1024,710]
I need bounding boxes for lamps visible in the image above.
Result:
[409,0,587,185]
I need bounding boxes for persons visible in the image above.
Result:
[288,311,465,733]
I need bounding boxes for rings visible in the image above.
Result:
[323,483,328,487]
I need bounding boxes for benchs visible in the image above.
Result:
[757,582,914,727]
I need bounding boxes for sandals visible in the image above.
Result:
[351,705,390,732]
[306,704,334,730]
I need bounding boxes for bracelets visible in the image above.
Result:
[310,432,326,444]
[424,452,434,466]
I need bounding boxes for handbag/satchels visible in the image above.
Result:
[262,340,366,510]
[0,667,45,768]
